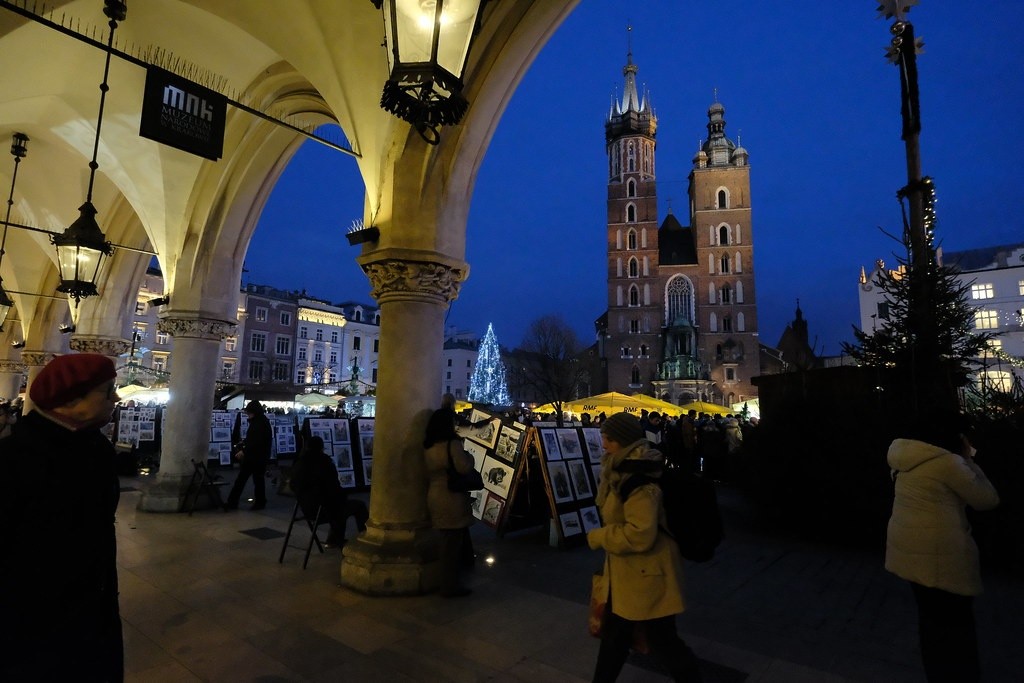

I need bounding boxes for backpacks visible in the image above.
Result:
[618,468,726,563]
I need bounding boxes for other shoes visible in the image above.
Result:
[249,502,266,510]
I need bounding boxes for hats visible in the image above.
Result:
[641,409,647,416]
[30,353,117,412]
[599,413,646,446]
[241,400,265,414]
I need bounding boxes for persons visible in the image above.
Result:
[424,409,475,596]
[0,398,757,464]
[588,413,705,683]
[226,401,273,512]
[0,354,123,683]
[290,436,369,548]
[884,422,1000,683]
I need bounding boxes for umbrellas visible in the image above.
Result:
[454,390,739,421]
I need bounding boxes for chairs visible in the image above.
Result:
[181,459,230,516]
[278,479,345,570]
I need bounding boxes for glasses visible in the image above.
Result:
[92,383,118,399]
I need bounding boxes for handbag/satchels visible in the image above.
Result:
[587,572,650,653]
[447,437,484,491]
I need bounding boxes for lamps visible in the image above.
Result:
[59,326,76,335]
[0,133,31,332]
[12,340,25,349]
[147,294,170,308]
[346,226,381,246]
[371,0,488,146]
[49,0,129,309]
